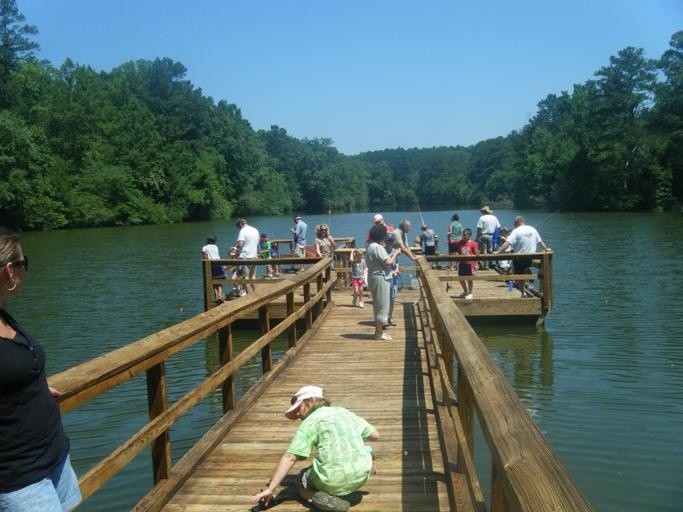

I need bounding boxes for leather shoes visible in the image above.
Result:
[217,300,223,304]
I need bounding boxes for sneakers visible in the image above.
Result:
[464,294,472,299]
[311,490,350,512]
[374,317,396,340]
[358,301,363,308]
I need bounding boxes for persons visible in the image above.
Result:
[289,217,307,271]
[350,214,421,341]
[421,223,436,269]
[448,206,551,299]
[251,385,381,512]
[224,218,280,297]
[315,223,336,298]
[0,233,84,512]
[202,235,226,305]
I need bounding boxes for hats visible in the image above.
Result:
[284,385,323,420]
[479,206,490,213]
[383,233,395,243]
[373,214,382,222]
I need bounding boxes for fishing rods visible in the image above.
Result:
[415,200,424,225]
[532,207,562,227]
[327,204,331,239]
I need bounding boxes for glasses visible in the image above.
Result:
[12,256,28,271]
[290,394,301,404]
